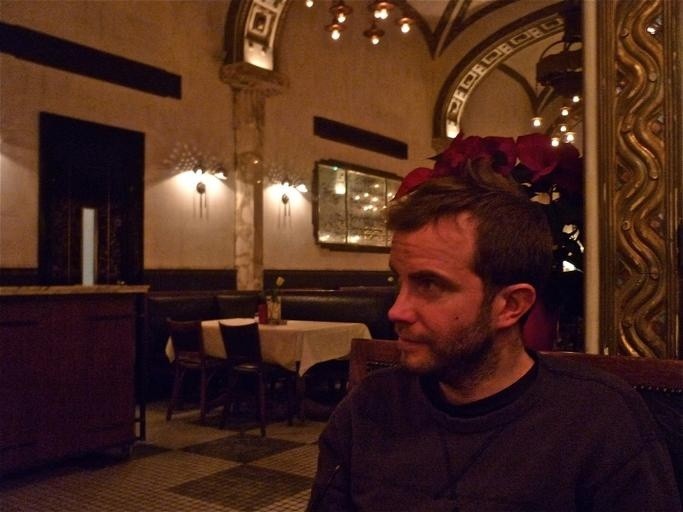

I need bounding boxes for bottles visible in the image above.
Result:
[258,303,268,324]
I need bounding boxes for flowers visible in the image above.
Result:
[270,276,285,320]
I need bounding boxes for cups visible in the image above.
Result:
[267,296,281,325]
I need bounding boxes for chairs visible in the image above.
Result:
[165,317,239,427]
[351,337,683,476]
[218,322,292,437]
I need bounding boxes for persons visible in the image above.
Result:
[303,158,681,509]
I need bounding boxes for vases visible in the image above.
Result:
[266,296,282,322]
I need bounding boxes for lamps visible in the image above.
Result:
[306,0,416,47]
[532,1,584,150]
